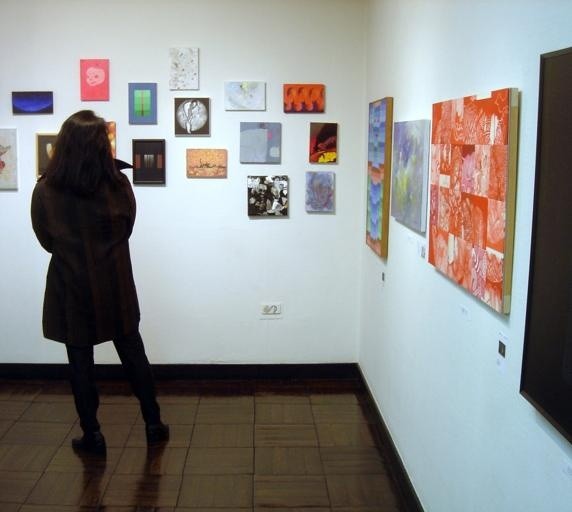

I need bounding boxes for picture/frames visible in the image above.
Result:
[133,138,167,184]
[34,131,62,180]
[365,97,393,261]
[173,95,214,139]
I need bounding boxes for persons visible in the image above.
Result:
[30,109,171,457]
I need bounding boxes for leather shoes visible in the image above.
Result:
[145,416,169,439]
[71,432,105,454]
